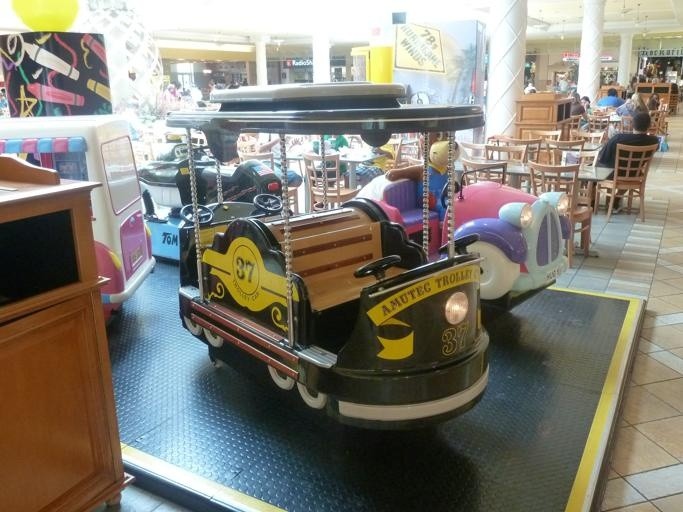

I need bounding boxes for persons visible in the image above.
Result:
[640,59,662,83]
[304,134,348,210]
[524,72,659,133]
[343,142,395,189]
[583,112,659,215]
[167,79,241,101]
[237,132,302,187]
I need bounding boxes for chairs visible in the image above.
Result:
[243,152,299,216]
[304,154,361,215]
[456,97,669,268]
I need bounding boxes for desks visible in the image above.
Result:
[338,153,381,190]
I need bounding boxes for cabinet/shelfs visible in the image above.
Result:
[635,82,678,115]
[0,152,137,511]
[598,84,627,100]
